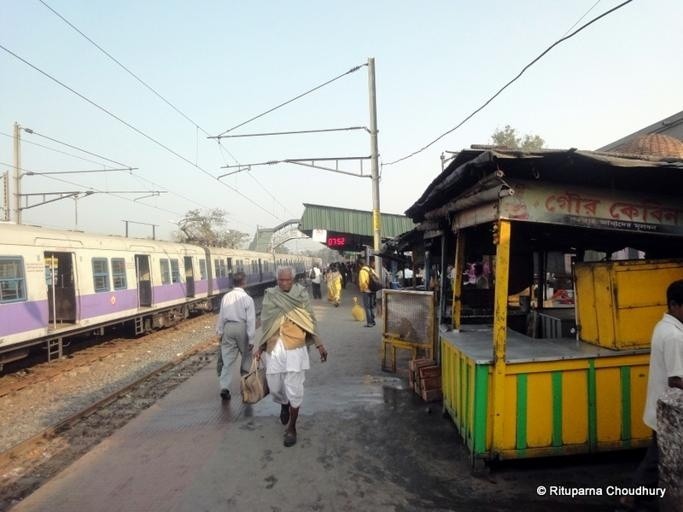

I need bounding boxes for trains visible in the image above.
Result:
[0,216,323,377]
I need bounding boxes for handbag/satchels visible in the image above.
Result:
[239,357,268,404]
[216,342,222,376]
[309,268,314,278]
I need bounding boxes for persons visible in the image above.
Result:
[252,264,328,446]
[309,251,493,329]
[214,271,257,402]
[618,279,683,511]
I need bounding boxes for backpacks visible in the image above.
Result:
[361,265,380,292]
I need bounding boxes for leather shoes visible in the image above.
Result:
[220,389,230,400]
[283,429,295,446]
[280,402,290,425]
